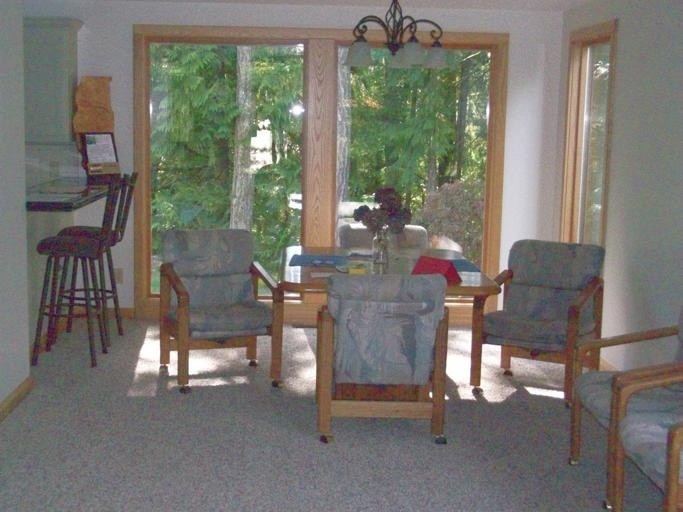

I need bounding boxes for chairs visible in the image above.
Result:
[31,171,140,369]
[568,305,682,511]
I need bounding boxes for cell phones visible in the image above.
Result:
[336,264,348,273]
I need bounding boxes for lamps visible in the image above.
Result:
[342,0,446,68]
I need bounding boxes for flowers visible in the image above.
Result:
[352,187,413,263]
